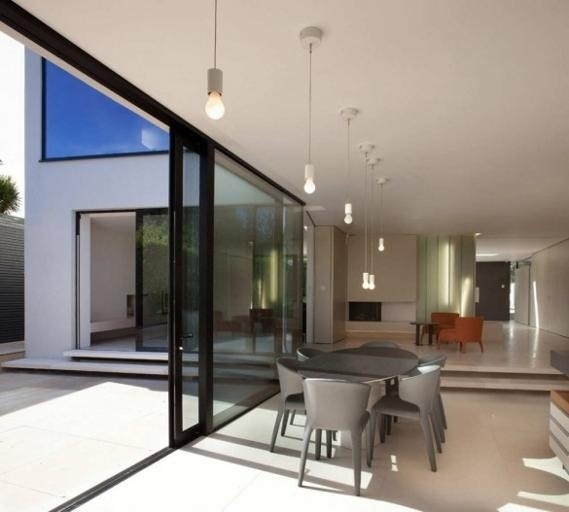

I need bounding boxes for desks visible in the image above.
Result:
[548,347,568,472]
[250,309,274,334]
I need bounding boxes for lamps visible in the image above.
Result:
[376,178,390,252]
[359,143,374,289]
[204,1,225,120]
[367,157,380,291]
[299,26,323,194]
[339,109,356,224]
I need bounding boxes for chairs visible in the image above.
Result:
[215,311,242,338]
[270,340,449,498]
[439,317,483,353]
[421,313,459,345]
